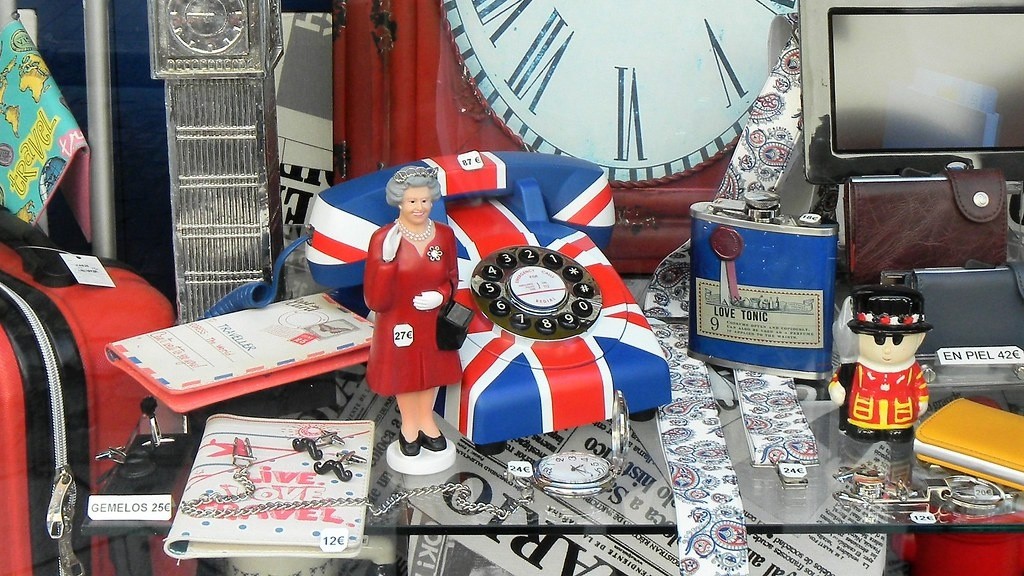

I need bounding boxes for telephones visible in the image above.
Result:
[305,155,673,454]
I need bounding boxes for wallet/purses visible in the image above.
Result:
[907,262,1024,348]
[912,396,1024,490]
[849,168,1010,275]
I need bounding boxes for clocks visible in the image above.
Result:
[332,0,797,272]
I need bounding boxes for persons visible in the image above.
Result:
[365,166,461,456]
[828,286,935,443]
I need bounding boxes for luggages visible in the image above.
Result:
[0,203,200,576]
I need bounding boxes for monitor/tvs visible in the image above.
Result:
[799,0,1024,183]
